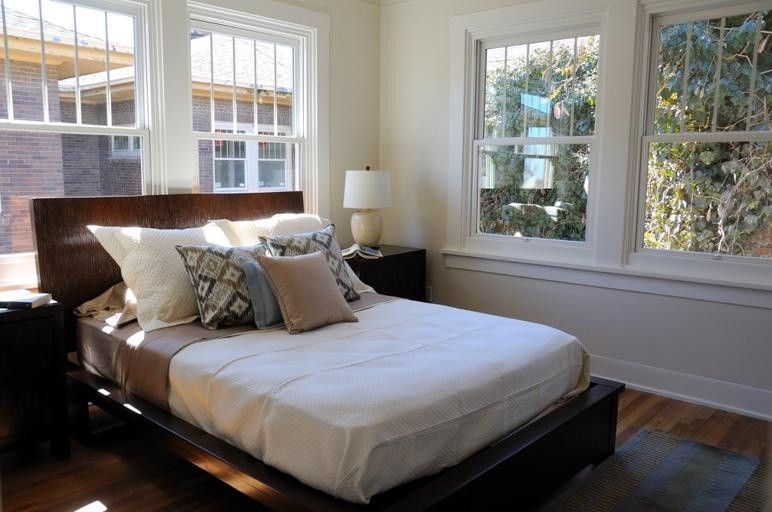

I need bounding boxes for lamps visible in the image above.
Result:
[342,165,392,246]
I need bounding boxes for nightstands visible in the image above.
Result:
[0,300,73,468]
[343,244,427,303]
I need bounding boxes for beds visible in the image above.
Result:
[32,191,627,512]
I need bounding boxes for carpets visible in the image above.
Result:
[541,427,772,512]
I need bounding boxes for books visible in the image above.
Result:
[341,242,383,260]
[0,292,53,311]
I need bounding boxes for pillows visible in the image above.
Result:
[72,280,138,330]
[257,224,360,303]
[174,244,260,329]
[86,223,218,334]
[236,255,286,331]
[256,251,359,336]
[207,212,331,248]
